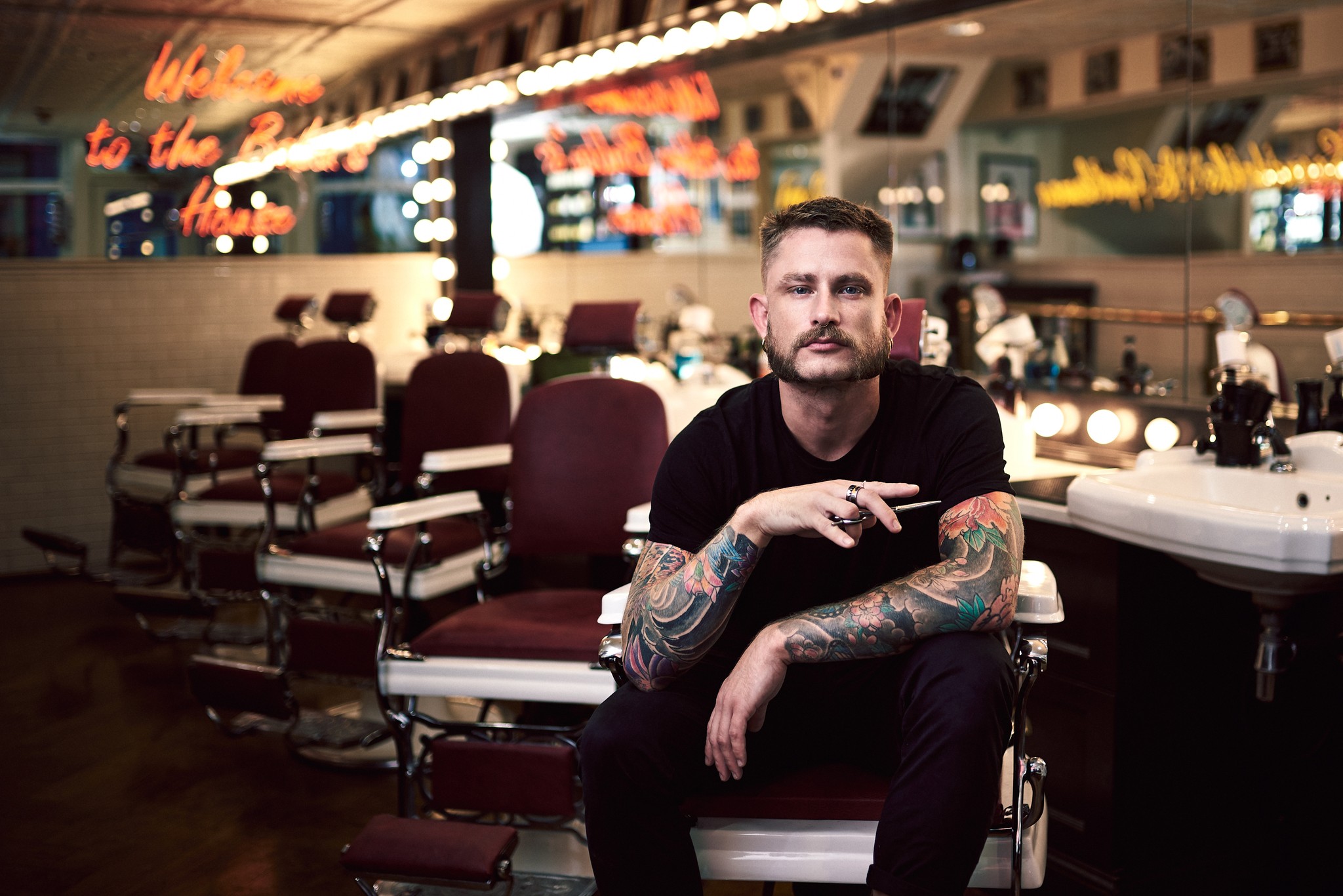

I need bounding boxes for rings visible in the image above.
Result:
[862,480,866,488]
[845,484,865,508]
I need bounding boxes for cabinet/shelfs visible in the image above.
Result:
[1022,514,1124,896]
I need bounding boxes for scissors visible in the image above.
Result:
[830,500,942,527]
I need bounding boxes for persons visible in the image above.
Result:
[578,194,1027,896]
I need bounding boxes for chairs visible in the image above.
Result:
[21,292,1066,896]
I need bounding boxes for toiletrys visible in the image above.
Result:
[1207,363,1261,468]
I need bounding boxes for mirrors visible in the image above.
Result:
[0,0,1343,460]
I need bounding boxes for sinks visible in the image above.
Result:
[1063,429,1343,564]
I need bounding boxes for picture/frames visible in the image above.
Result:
[978,152,1041,246]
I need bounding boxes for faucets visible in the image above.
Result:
[1247,424,1296,473]
